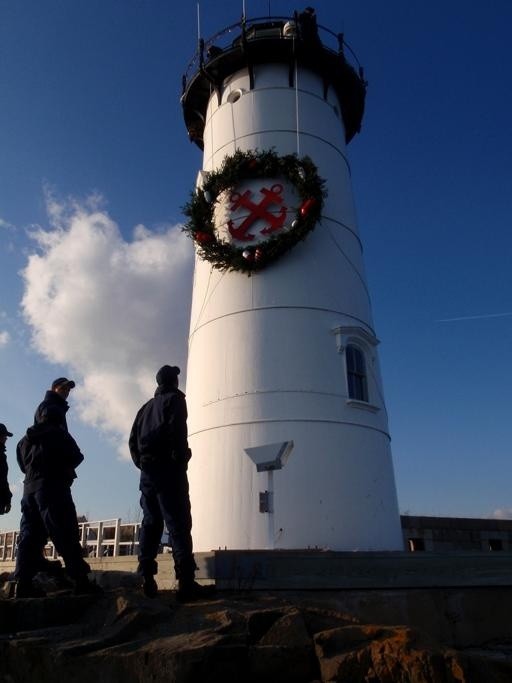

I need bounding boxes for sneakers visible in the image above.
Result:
[175,579,215,595]
[142,575,158,597]
[68,576,91,592]
[14,583,47,599]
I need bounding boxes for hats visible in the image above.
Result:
[0,423,13,437]
[52,378,75,388]
[156,365,180,385]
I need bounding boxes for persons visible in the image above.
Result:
[0,423,14,515]
[128,364,207,601]
[11,377,102,599]
